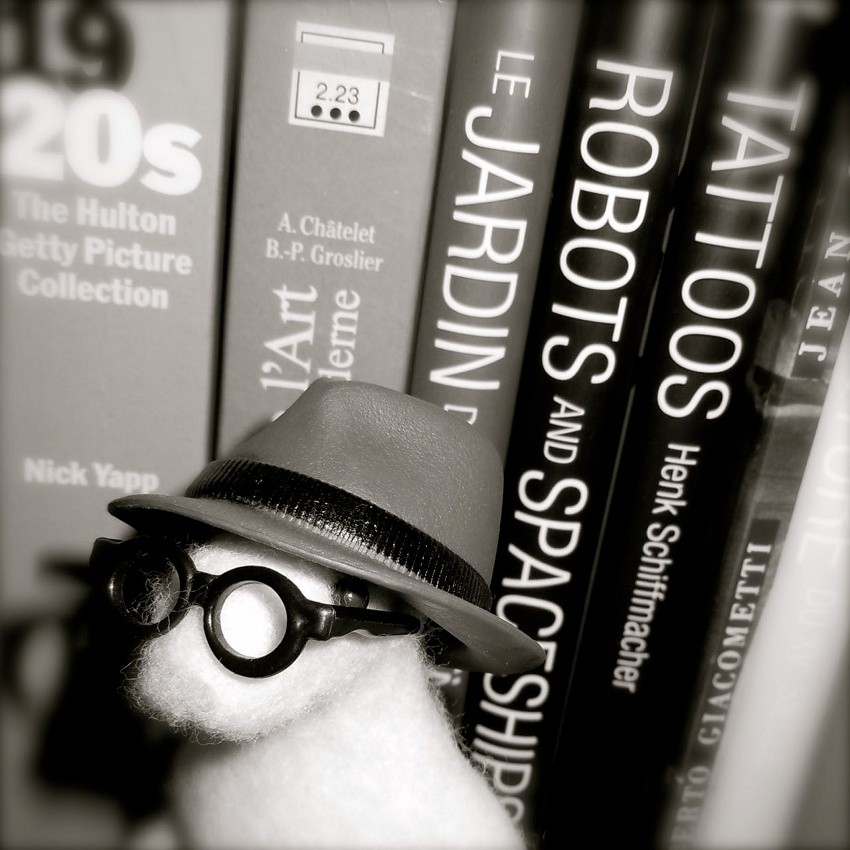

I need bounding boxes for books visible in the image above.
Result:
[2,0,849,850]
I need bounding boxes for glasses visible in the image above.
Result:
[87,537,421,679]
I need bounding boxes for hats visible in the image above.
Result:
[107,378,546,675]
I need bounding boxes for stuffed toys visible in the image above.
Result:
[82,377,532,850]
